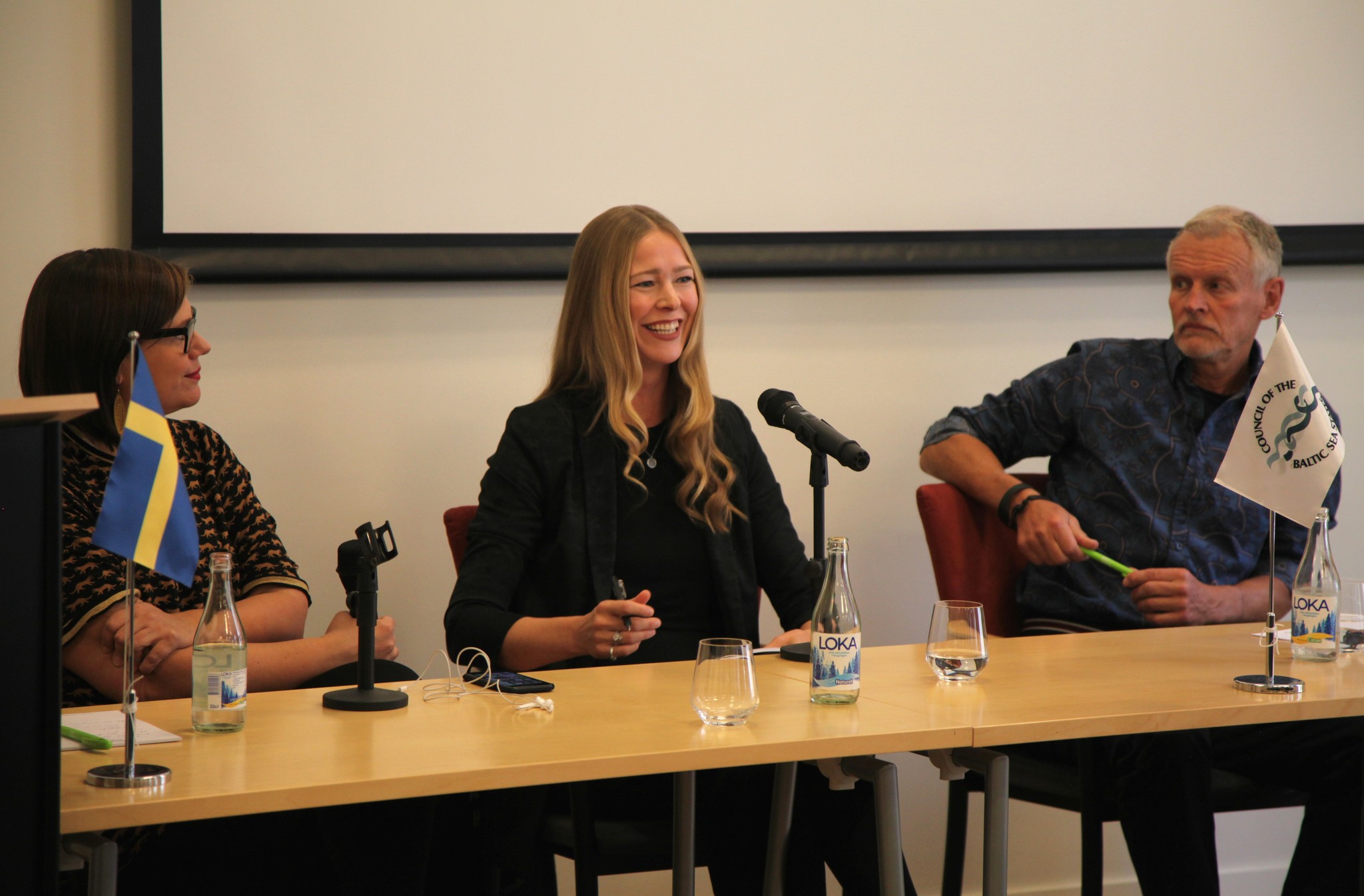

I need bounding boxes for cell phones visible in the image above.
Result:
[464,671,554,694]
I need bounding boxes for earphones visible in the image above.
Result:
[516,696,554,712]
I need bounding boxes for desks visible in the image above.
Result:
[72,611,1364,896]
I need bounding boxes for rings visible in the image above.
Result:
[612,631,623,646]
[609,647,617,661]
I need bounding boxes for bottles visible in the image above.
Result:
[1291,507,1341,662]
[809,536,861,705]
[192,552,248,735]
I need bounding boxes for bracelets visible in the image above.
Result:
[998,482,1033,524]
[1011,494,1048,532]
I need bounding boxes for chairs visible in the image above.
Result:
[445,505,709,896]
[916,473,1305,896]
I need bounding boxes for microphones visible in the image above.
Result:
[336,539,379,625]
[758,388,870,472]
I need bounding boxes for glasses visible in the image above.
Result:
[138,306,197,354]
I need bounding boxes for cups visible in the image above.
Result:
[1337,579,1364,652]
[691,638,760,726]
[926,600,989,682]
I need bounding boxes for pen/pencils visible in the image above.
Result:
[1079,547,1133,577]
[61,725,113,750]
[610,574,632,632]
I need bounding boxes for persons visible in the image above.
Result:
[442,203,920,895]
[16,247,399,708]
[914,201,1364,896]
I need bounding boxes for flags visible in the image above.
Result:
[91,340,200,588]
[1214,316,1345,530]
